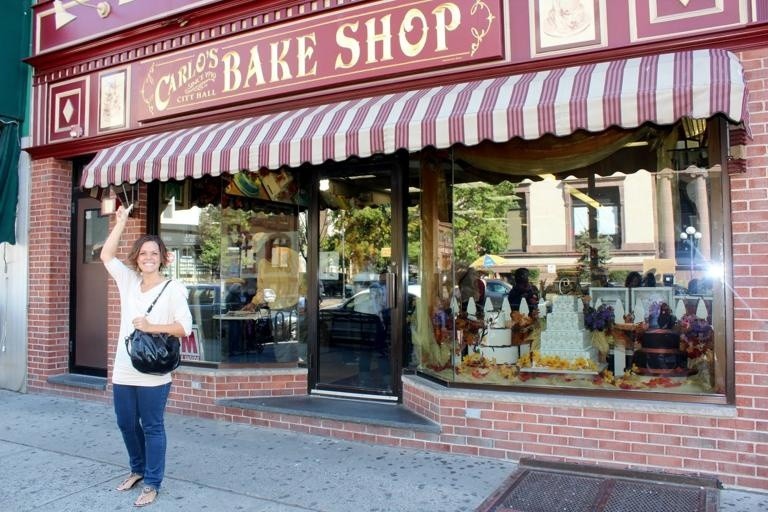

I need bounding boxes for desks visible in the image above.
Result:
[211,314,271,357]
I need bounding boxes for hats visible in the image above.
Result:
[251,232,268,254]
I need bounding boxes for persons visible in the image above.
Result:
[444,260,481,357]
[240,231,309,364]
[624,271,643,288]
[226,282,247,358]
[348,259,394,396]
[97,203,195,506]
[368,268,391,356]
[506,267,539,317]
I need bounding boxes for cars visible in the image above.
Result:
[319,286,421,353]
[184,283,225,339]
[450,274,513,311]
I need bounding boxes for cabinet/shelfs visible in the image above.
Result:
[588,286,675,356]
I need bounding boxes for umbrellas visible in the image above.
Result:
[466,254,510,279]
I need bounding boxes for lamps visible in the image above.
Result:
[52,0,111,32]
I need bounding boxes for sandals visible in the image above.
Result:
[116,473,144,490]
[133,486,157,507]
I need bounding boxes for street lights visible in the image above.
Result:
[681,225,701,287]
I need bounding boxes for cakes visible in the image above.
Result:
[467,311,518,365]
[630,313,688,373]
[533,295,599,370]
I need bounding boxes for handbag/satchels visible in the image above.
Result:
[125,329,182,376]
